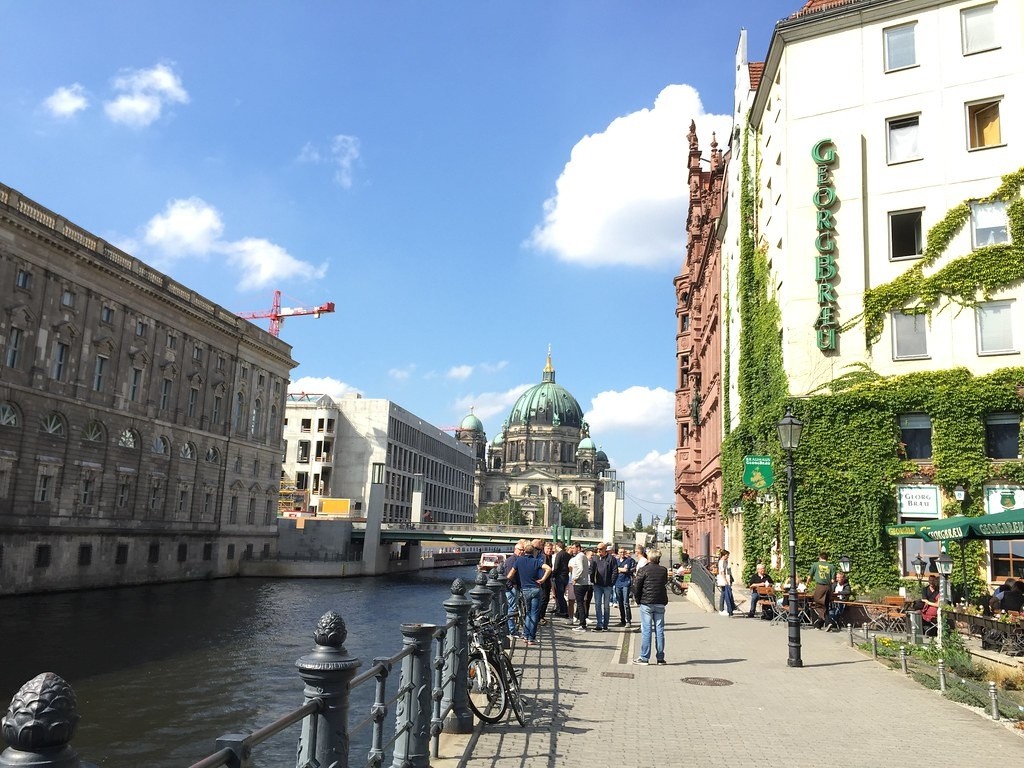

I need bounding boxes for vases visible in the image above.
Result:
[943,610,1024,634]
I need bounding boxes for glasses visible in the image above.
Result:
[518,548,525,551]
[596,548,602,551]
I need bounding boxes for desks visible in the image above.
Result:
[862,601,900,632]
[782,593,814,625]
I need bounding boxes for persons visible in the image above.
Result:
[828,571,851,618]
[989,578,1024,615]
[682,548,691,565]
[716,550,737,616]
[633,550,669,665]
[805,552,835,632]
[785,575,807,592]
[912,574,941,634]
[504,537,652,645]
[747,564,777,618]
[423,510,433,522]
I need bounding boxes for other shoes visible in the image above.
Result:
[718,610,729,616]
[633,656,649,666]
[564,621,573,625]
[625,623,631,628]
[657,659,666,665]
[824,624,832,632]
[549,609,558,613]
[586,618,594,624]
[527,640,542,645]
[603,627,608,631]
[555,612,569,619]
[591,627,603,632]
[572,625,587,632]
[615,622,625,627]
[512,631,522,636]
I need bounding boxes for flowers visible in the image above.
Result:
[942,602,1024,625]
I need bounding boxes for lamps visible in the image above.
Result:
[837,556,855,573]
[911,553,927,580]
[934,553,954,578]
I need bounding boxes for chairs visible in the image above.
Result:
[758,583,846,630]
[885,596,938,637]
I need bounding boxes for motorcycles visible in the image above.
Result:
[667,573,692,596]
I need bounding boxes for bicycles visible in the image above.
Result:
[466,607,527,729]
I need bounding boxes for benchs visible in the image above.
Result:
[834,601,887,630]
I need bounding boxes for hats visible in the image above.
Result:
[597,542,607,548]
[605,546,613,551]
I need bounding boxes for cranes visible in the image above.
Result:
[232,290,336,339]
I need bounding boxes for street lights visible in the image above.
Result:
[654,514,661,550]
[666,504,678,572]
[777,405,807,667]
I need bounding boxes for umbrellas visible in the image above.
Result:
[922,508,1024,543]
[885,512,973,606]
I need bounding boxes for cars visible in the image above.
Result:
[673,553,718,575]
